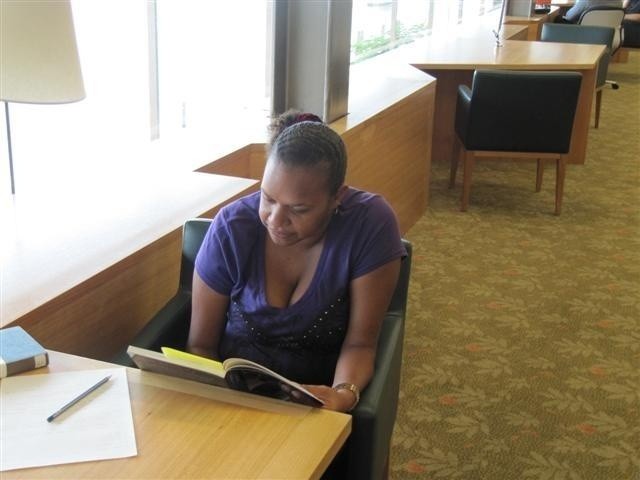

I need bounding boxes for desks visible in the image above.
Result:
[410,37,607,167]
[0,342,353,479]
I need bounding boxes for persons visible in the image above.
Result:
[554,0,640,35]
[187,108,408,412]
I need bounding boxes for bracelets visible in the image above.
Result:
[333,383,360,411]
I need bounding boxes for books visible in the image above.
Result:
[126,345,326,408]
[0,325,49,379]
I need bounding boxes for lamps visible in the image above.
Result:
[0,0,86,196]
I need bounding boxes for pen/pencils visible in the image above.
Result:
[48,375,112,421]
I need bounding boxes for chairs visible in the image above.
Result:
[449,5,631,219]
[117,218,412,479]
